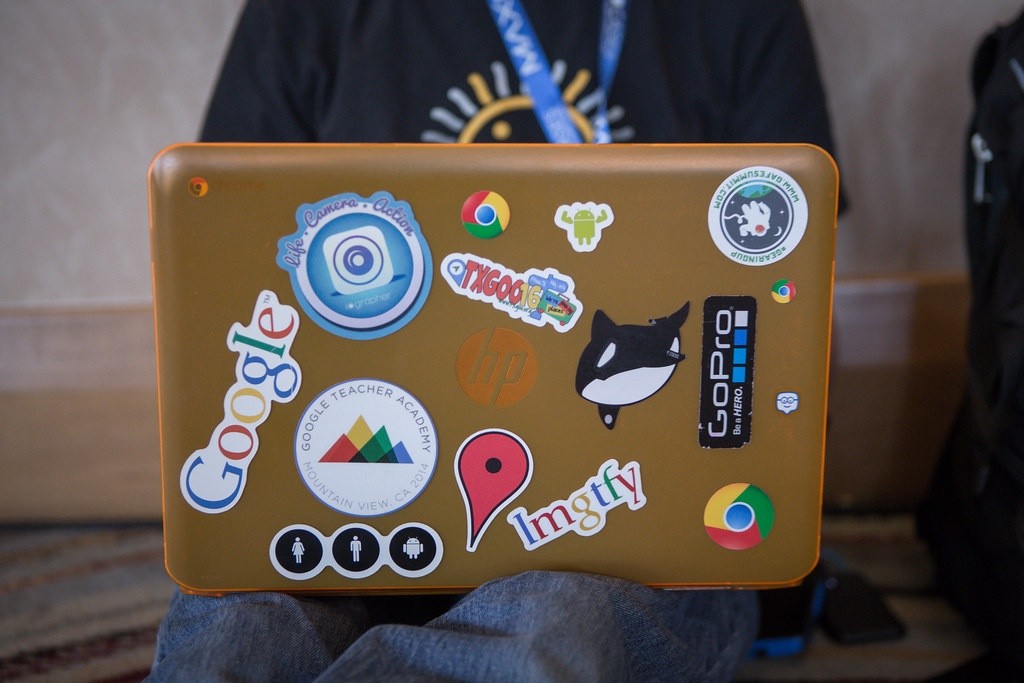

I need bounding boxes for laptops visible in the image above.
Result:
[148,141,840,595]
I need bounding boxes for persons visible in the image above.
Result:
[915,12,1024,655]
[141,0,848,683]
